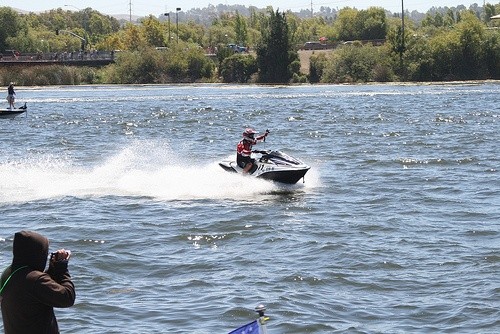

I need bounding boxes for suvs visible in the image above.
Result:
[303,41,327,50]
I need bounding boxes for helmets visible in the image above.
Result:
[243,129,259,143]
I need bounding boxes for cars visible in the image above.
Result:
[229,44,248,53]
[336,40,353,46]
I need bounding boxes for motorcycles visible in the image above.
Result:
[218,148,312,184]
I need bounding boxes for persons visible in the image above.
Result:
[237,128,268,174]
[8,82,16,109]
[0,230,75,334]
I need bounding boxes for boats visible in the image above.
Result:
[0,101,28,119]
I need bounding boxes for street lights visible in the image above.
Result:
[176,7,182,44]
[64,4,85,53]
[164,12,170,42]
